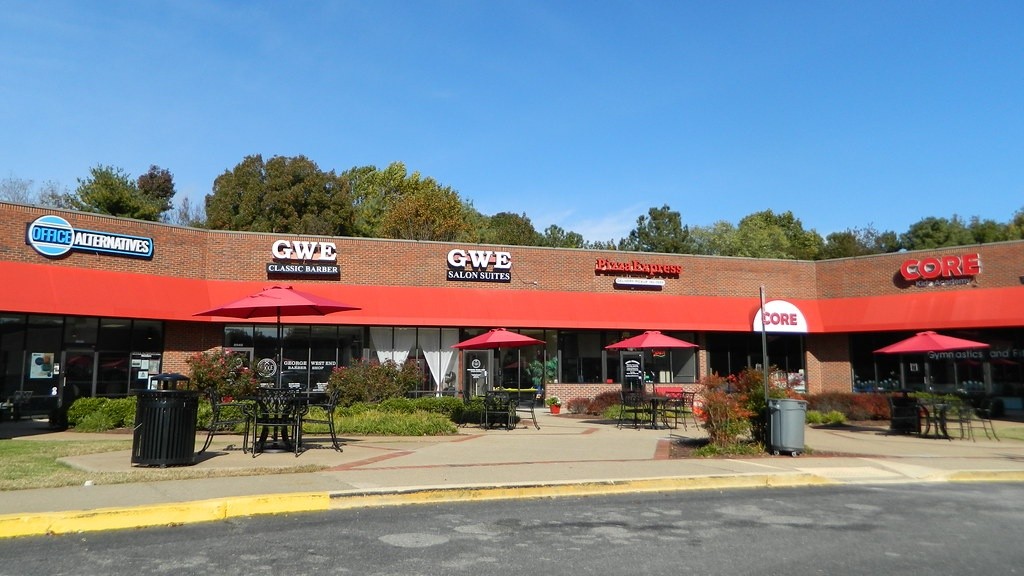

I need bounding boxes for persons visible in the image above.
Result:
[193,285,362,449]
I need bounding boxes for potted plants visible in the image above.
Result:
[544,396,562,414]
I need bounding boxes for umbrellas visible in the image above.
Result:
[873,332,989,434]
[605,331,699,426]
[451,328,546,427]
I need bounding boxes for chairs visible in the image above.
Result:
[457,388,540,431]
[191,371,343,457]
[615,377,700,432]
[886,392,1000,441]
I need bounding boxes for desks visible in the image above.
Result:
[920,398,951,440]
[486,390,519,430]
[642,397,673,429]
[248,392,326,452]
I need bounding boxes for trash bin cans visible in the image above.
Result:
[766,399,809,457]
[128,373,199,467]
